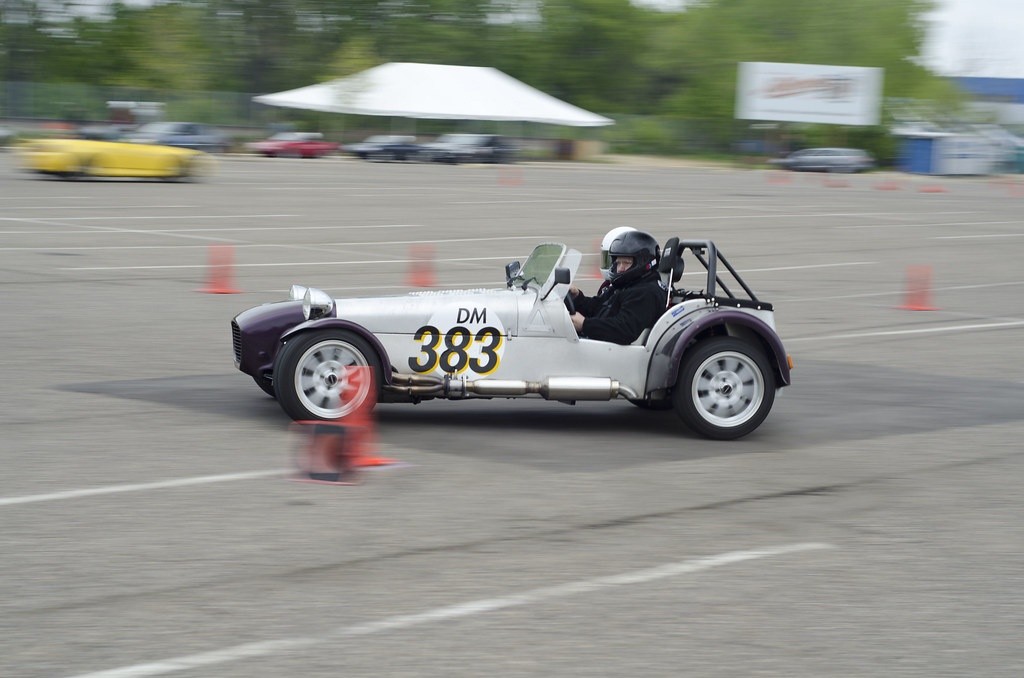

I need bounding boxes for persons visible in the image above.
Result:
[570,227,669,345]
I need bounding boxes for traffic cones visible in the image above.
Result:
[897,259,936,308]
[194,246,239,293]
[408,245,433,286]
[332,366,392,469]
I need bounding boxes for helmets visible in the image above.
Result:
[607,231,661,288]
[599,226,640,281]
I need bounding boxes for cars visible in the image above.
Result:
[16,121,510,182]
[769,145,874,172]
